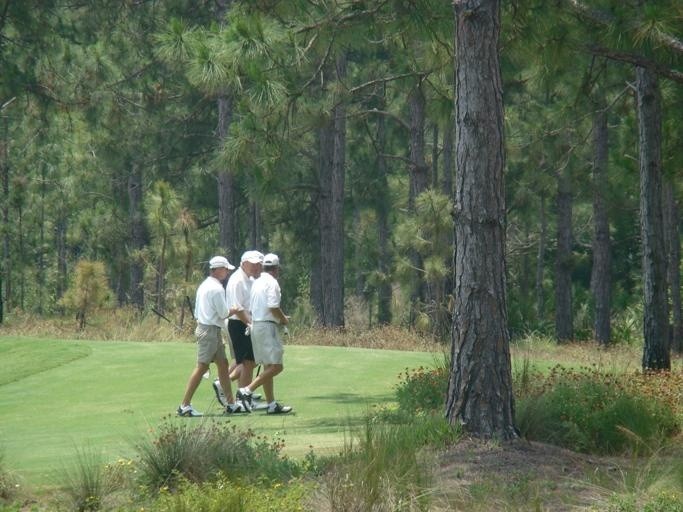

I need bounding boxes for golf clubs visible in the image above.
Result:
[283,314,292,336]
[186,296,210,379]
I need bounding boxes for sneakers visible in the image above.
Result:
[267,400,293,414]
[211,379,226,405]
[224,388,267,413]
[174,403,202,417]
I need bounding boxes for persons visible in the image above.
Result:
[175,250,292,417]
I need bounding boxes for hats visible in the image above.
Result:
[209,256,234,270]
[263,254,278,267]
[239,251,263,265]
